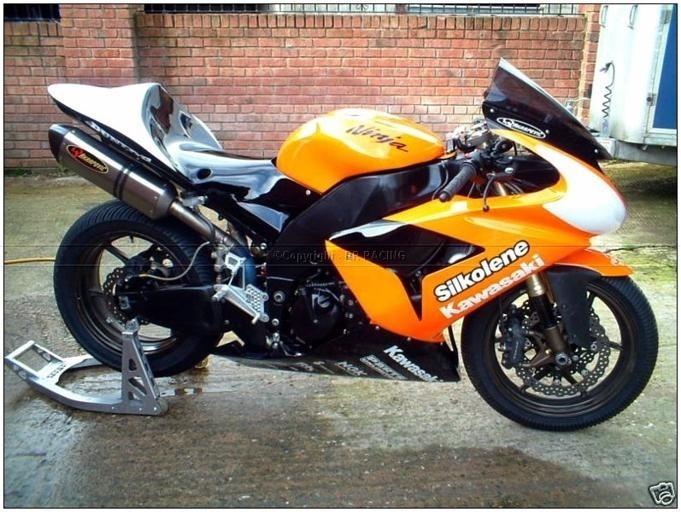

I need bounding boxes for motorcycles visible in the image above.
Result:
[43,59,661,435]
